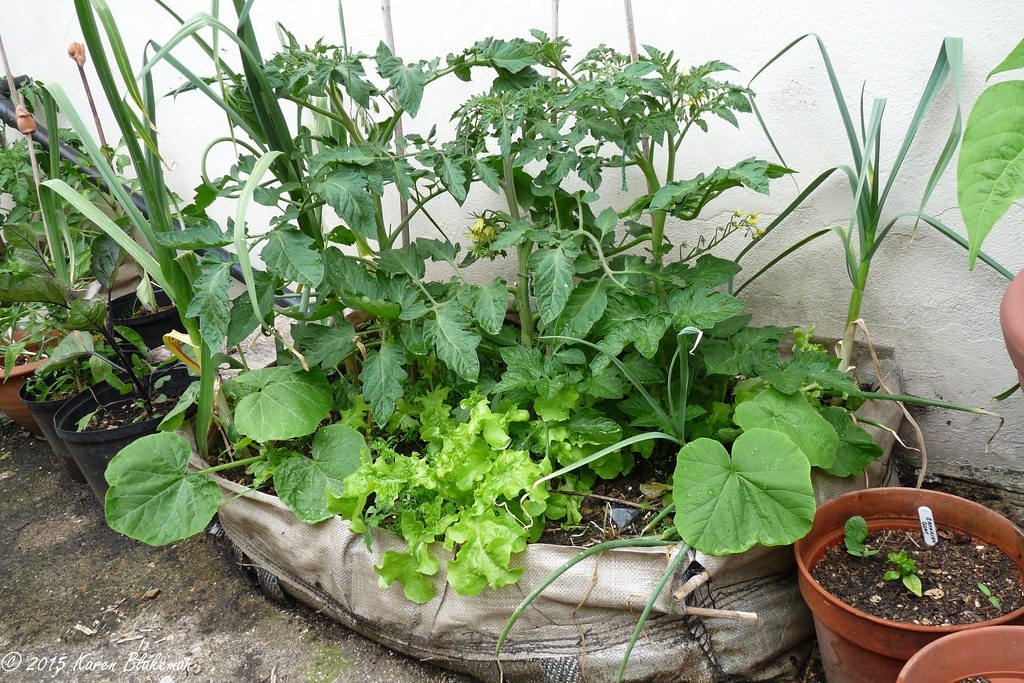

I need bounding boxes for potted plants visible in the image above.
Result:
[0,122,232,528]
[794,486,1024,683]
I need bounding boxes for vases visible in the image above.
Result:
[894,624,1024,683]
